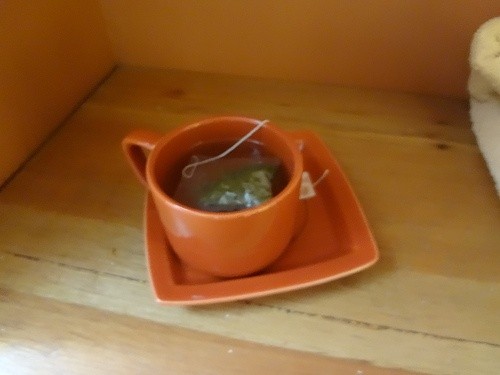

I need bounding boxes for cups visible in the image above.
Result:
[122,115,304,277]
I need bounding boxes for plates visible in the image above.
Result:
[144,131,380,306]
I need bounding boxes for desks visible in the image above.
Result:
[0,62,500,375]
